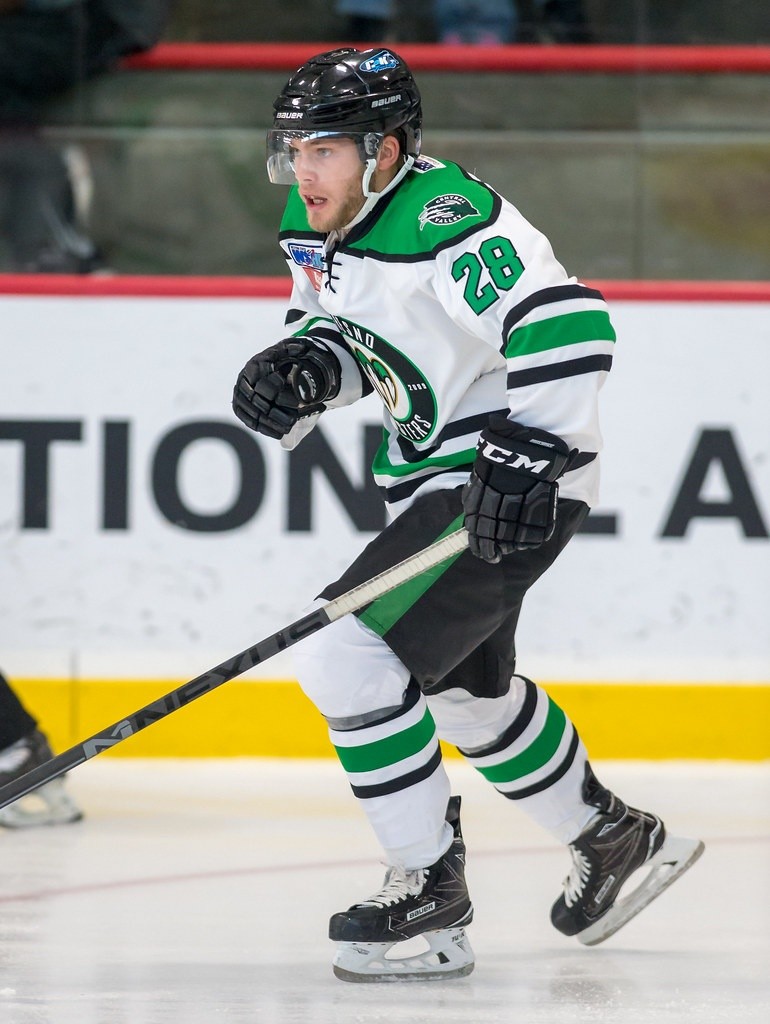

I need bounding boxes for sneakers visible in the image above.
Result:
[0,731,85,828]
[328,797,478,983]
[551,760,703,946]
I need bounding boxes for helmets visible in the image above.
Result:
[274,48,422,154]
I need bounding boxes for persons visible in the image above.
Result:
[233,49,706,983]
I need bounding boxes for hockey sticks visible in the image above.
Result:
[1,498,593,811]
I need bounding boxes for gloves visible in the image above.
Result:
[234,338,331,440]
[461,419,567,563]
